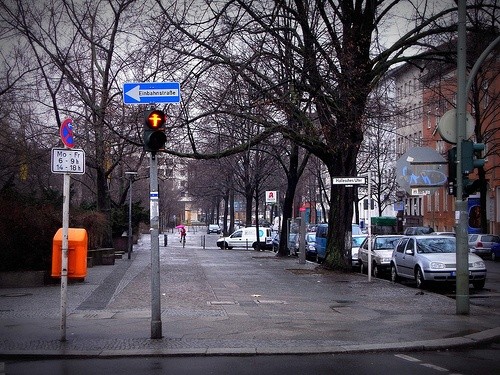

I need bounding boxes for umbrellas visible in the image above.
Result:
[176,225,184,228]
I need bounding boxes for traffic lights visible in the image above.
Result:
[144,110,167,151]
[461,140,485,175]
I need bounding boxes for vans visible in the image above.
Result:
[217,225,272,251]
[315,223,362,264]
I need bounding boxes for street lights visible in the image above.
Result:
[125,171,138,260]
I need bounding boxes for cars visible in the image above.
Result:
[404,227,434,236]
[351,234,369,267]
[390,235,487,290]
[305,232,316,258]
[207,224,221,234]
[430,231,455,236]
[272,233,296,253]
[467,234,500,255]
[358,234,407,278]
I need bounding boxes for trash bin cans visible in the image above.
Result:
[51,227,88,279]
[112,235,133,252]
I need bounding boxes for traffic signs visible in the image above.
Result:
[123,82,180,102]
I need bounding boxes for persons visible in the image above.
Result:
[179,227,186,243]
[426,225,434,234]
